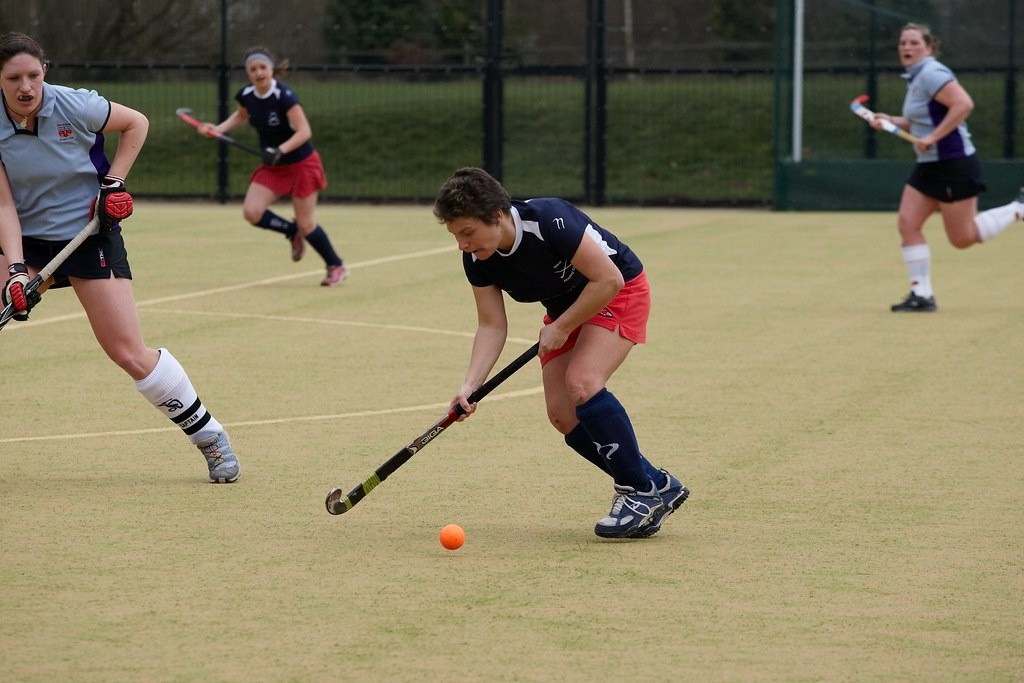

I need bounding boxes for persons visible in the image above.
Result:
[197,47,351,287]
[433,167,691,539]
[0,33,241,483]
[868,23,1024,312]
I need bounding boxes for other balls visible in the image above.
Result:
[440,524,466,550]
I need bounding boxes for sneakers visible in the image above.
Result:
[321,260,349,285]
[891,291,936,312]
[196,429,241,483]
[595,479,664,538]
[1015,186,1024,203]
[290,227,304,261]
[629,468,690,540]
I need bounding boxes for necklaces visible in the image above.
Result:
[8,105,39,128]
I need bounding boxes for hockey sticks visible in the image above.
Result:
[176,106,265,161]
[0,212,100,332]
[325,342,539,517]
[850,93,932,152]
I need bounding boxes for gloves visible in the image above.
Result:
[89,174,133,238]
[263,146,283,166]
[2,263,42,320]
